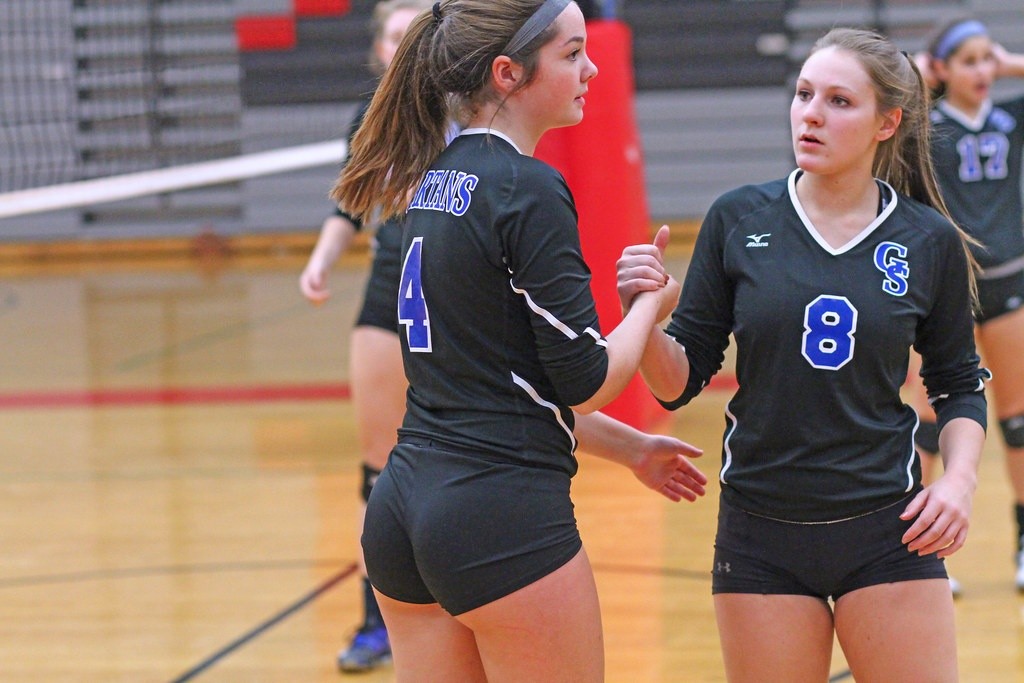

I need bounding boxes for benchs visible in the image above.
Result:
[240,0,1024,226]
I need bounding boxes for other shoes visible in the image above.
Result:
[950,579,959,592]
[1015,545,1024,590]
[337,619,393,674]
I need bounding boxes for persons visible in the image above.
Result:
[323,0,705,682]
[901,21,1024,596]
[299,0,462,673]
[619,26,992,683]
